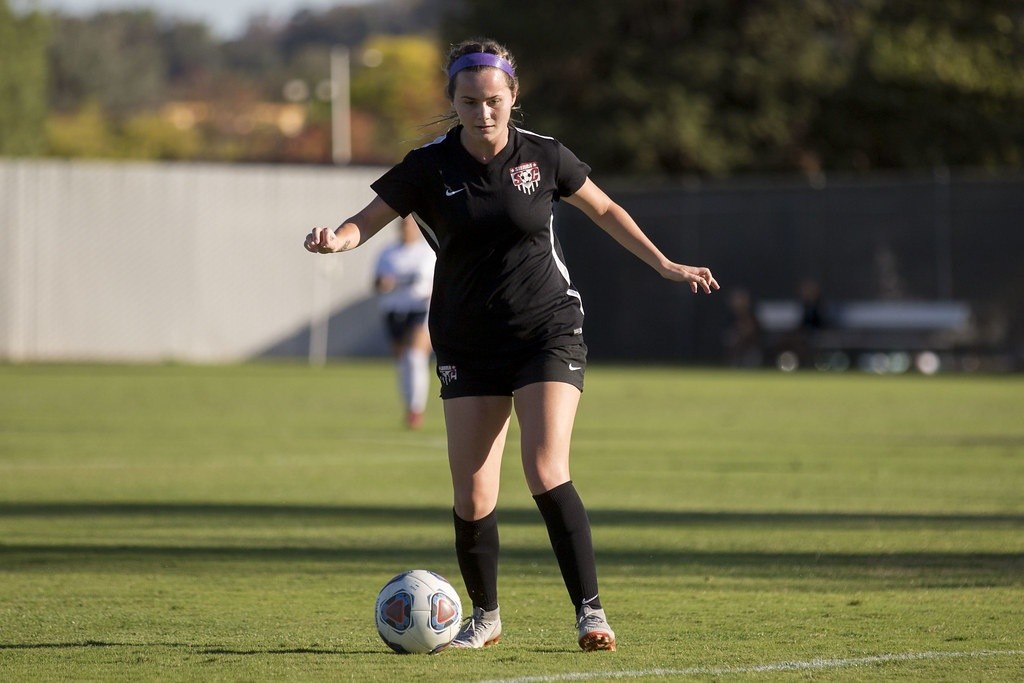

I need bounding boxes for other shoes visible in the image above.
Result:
[407,408,424,429]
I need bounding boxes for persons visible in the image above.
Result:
[728,279,837,372]
[303,41,720,651]
[372,212,437,429]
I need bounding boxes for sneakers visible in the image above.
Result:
[575,605,616,652]
[448,602,502,650]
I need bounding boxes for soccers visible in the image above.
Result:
[375,569,463,655]
